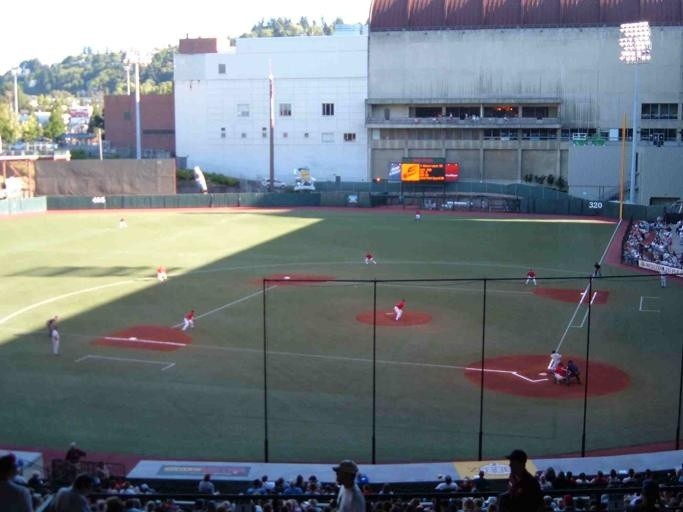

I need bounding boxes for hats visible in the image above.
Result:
[505,449,528,460]
[333,460,358,474]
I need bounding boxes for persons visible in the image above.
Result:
[1,444,683,512]
[594,261,603,280]
[157,262,168,284]
[364,250,377,265]
[393,298,406,321]
[181,310,194,332]
[622,215,682,288]
[46,315,60,356]
[413,206,422,223]
[546,350,582,387]
[523,268,536,287]
[398,191,405,204]
[119,216,127,229]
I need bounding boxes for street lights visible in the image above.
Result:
[10,65,19,114]
[121,58,132,97]
[265,72,274,192]
[131,49,141,158]
[95,126,106,161]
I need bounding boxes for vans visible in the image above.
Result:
[294,180,315,193]
[53,149,71,161]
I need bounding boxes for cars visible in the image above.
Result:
[261,177,287,190]
[495,133,508,140]
